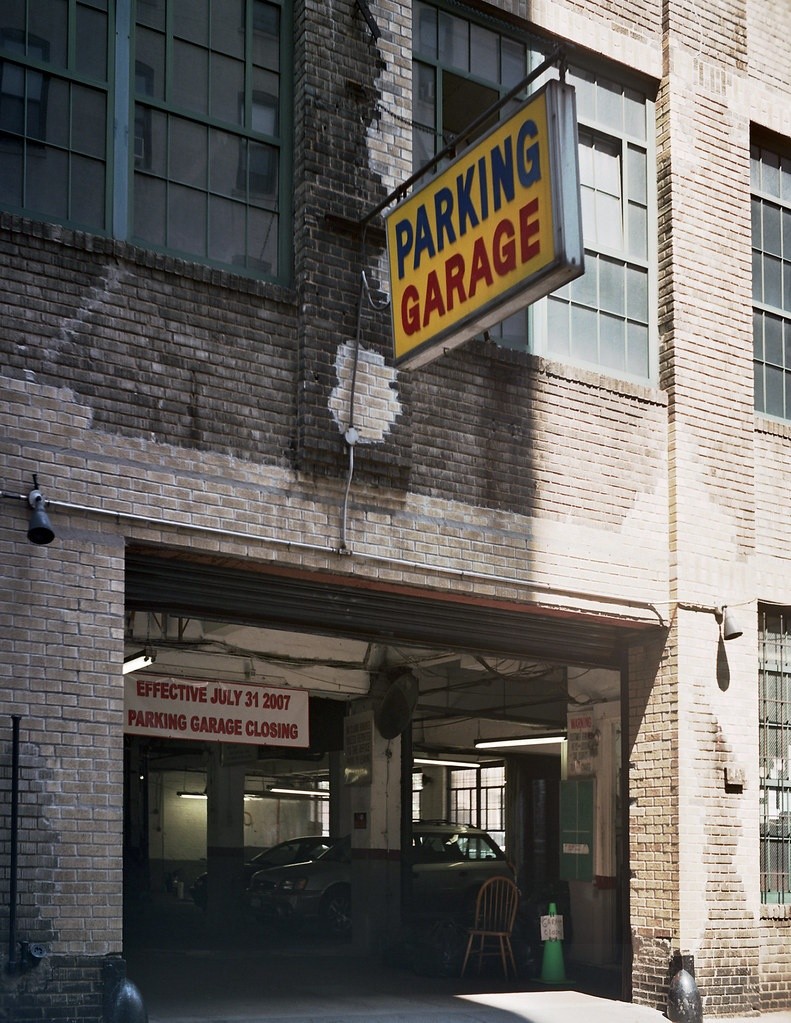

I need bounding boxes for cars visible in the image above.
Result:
[169,835,332,911]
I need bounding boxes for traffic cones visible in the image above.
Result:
[531,903,575,984]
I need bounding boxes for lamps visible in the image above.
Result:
[473,716,567,749]
[27,490,56,545]
[413,752,480,768]
[717,602,743,640]
[266,785,330,796]
[176,771,265,800]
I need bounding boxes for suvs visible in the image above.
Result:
[248,818,510,940]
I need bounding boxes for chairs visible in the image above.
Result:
[458,877,520,979]
[284,846,297,863]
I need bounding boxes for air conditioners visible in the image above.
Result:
[133,136,144,165]
